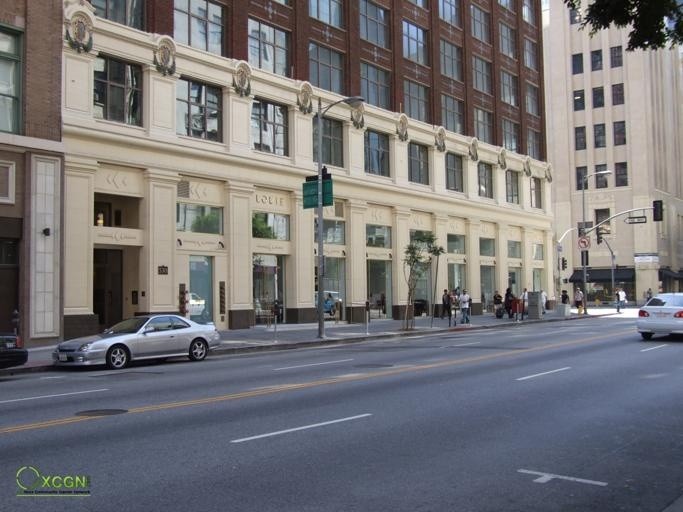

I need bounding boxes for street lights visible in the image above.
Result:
[318,95,365,338]
[582,171,613,316]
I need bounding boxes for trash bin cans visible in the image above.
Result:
[414,299,427,316]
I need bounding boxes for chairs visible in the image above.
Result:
[369,293,385,317]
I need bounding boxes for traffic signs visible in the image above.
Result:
[624,216,647,224]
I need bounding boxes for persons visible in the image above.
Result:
[492,289,503,317]
[504,287,515,319]
[575,287,584,314]
[519,287,528,316]
[614,288,621,313]
[561,289,570,305]
[439,286,470,324]
[538,288,548,314]
[594,291,602,307]
[270,301,280,324]
[646,288,652,302]
[618,287,626,309]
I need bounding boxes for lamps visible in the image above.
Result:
[480,260,496,264]
[447,257,467,263]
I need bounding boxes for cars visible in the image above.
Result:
[52,314,220,369]
[0,336,27,368]
[638,293,683,339]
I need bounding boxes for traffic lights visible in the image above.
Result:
[562,257,567,270]
[597,226,603,244]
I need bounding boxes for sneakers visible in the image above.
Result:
[461,318,470,324]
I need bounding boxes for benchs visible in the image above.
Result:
[255,308,282,328]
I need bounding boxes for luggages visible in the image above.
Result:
[496,307,505,318]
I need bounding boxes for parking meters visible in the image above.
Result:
[271,299,282,337]
[365,301,369,333]
[11,308,19,335]
[469,298,472,323]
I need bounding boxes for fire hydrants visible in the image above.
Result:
[579,306,583,316]
